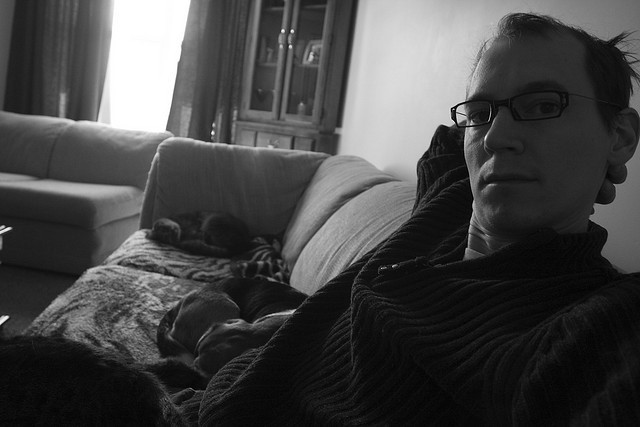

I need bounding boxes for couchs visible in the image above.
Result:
[20,136,417,397]
[0,110,174,277]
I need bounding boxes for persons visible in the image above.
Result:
[166,12,640,426]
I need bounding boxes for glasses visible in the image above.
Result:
[451,89,622,128]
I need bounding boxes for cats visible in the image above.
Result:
[157,278,309,377]
[149,210,250,261]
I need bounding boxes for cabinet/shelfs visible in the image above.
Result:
[231,0,352,154]
